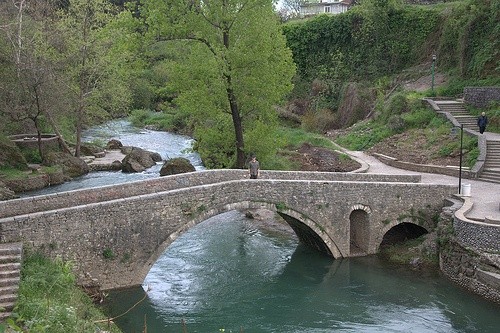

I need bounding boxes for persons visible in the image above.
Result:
[477,112,488,134]
[248,155,260,179]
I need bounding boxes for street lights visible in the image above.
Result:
[452,123,462,194]
[430,54,436,91]
